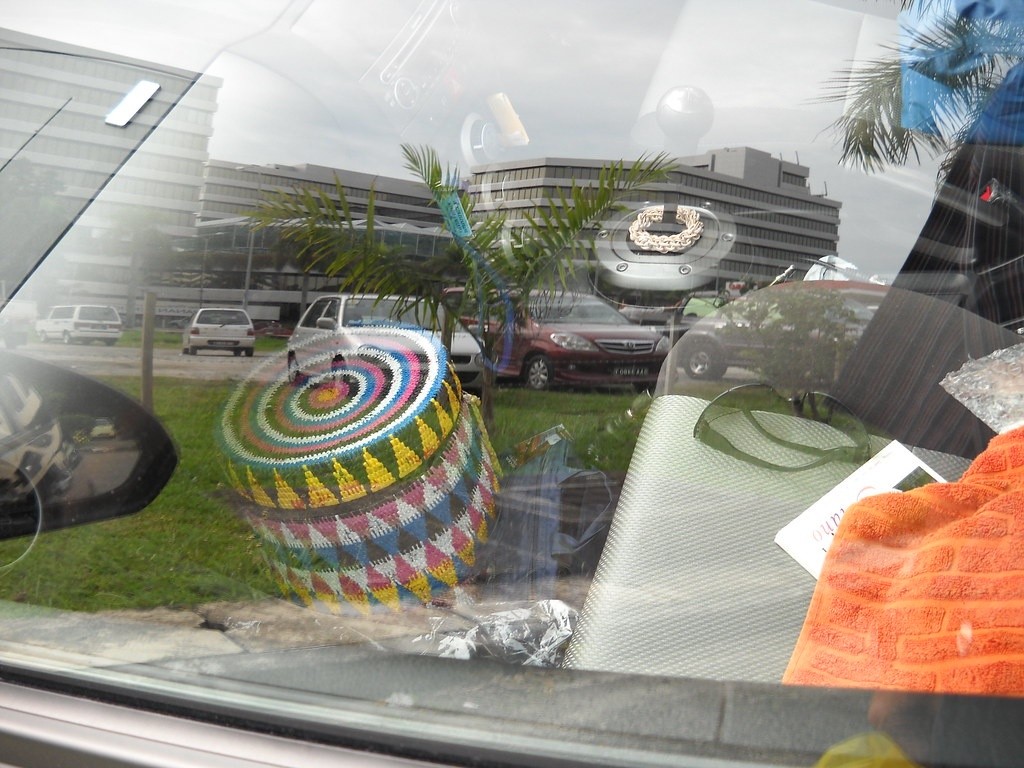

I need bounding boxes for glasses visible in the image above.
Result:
[693,383,871,473]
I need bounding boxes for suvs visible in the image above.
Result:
[35,303,123,347]
[615,289,875,388]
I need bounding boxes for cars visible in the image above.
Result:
[89,418,116,440]
[286,293,483,389]
[181,308,256,356]
[441,285,674,393]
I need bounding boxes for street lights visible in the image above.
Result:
[192,231,225,308]
[235,163,279,313]
